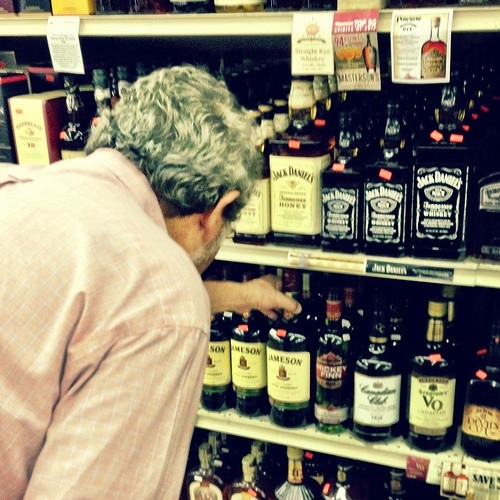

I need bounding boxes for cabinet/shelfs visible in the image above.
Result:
[0,5,500,500]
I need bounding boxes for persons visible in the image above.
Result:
[0,63,302,500]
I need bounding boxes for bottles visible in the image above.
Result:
[421,17,446,78]
[61,54,500,261]
[195,260,500,462]
[442,463,456,496]
[363,35,377,73]
[455,464,469,498]
[177,429,406,500]
